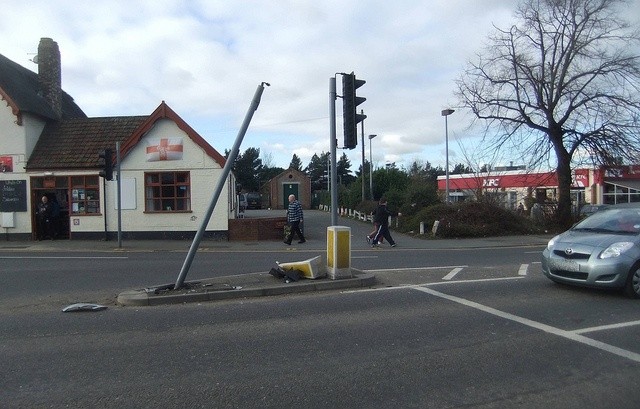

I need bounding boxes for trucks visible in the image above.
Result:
[247,192,262,209]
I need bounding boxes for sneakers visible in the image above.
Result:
[366,235,371,244]
[297,240,305,243]
[371,245,379,248]
[392,243,397,247]
[284,240,291,244]
[377,241,383,245]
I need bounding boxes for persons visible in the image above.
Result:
[283,194,306,245]
[48,192,61,237]
[366,202,384,244]
[35,195,52,241]
[371,198,398,249]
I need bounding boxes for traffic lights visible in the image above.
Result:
[354,74,368,145]
[99,150,106,178]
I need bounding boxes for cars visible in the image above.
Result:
[579,204,609,219]
[240,194,248,210]
[539,200,640,299]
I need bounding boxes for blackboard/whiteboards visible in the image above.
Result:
[0,180,26,211]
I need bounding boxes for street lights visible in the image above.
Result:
[369,134,377,201]
[442,108,456,205]
